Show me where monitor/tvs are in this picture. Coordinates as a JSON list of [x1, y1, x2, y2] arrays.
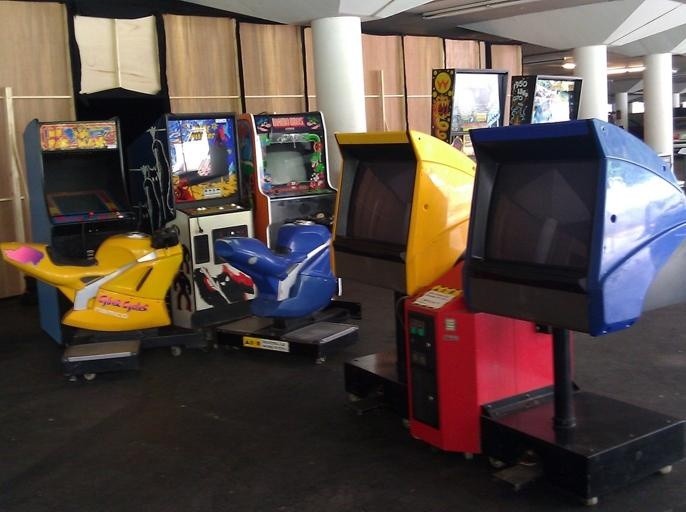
[[484, 157, 598, 272], [266, 142, 310, 185], [168, 118, 240, 204], [451, 72, 503, 132], [52, 192, 111, 217], [531, 79, 576, 126], [346, 158, 416, 247]]
